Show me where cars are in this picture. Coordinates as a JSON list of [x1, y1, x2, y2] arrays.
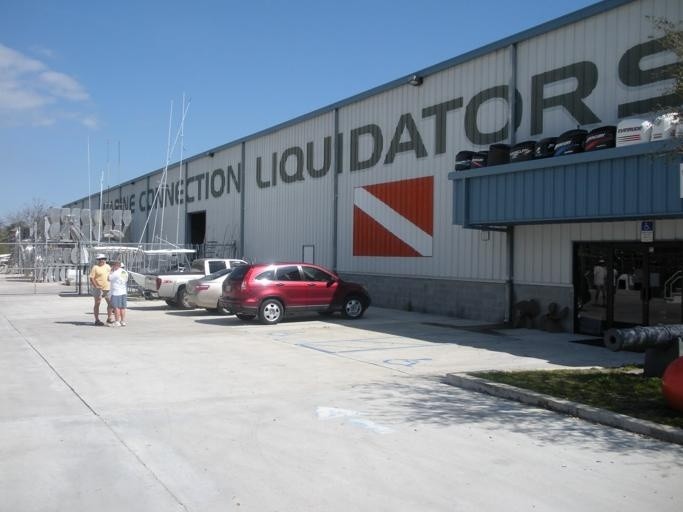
[[188, 266, 237, 316], [219, 261, 373, 325]]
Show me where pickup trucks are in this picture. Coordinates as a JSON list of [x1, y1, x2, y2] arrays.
[[144, 258, 248, 309]]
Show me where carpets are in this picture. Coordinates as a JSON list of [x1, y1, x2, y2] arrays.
[[569, 339, 646, 353]]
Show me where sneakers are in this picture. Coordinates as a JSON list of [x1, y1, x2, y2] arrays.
[[94, 320, 104, 326], [107, 318, 127, 327]]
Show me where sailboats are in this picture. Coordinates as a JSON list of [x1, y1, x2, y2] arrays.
[[127, 91, 197, 300]]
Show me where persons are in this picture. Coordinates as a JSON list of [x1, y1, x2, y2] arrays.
[[89, 254, 114, 326], [108, 261, 128, 327], [593, 259, 619, 307]]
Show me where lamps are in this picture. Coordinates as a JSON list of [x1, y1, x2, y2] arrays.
[[206, 152, 214, 157], [408, 75, 423, 86]]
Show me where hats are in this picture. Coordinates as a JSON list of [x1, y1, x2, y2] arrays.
[[96, 254, 107, 260]]
